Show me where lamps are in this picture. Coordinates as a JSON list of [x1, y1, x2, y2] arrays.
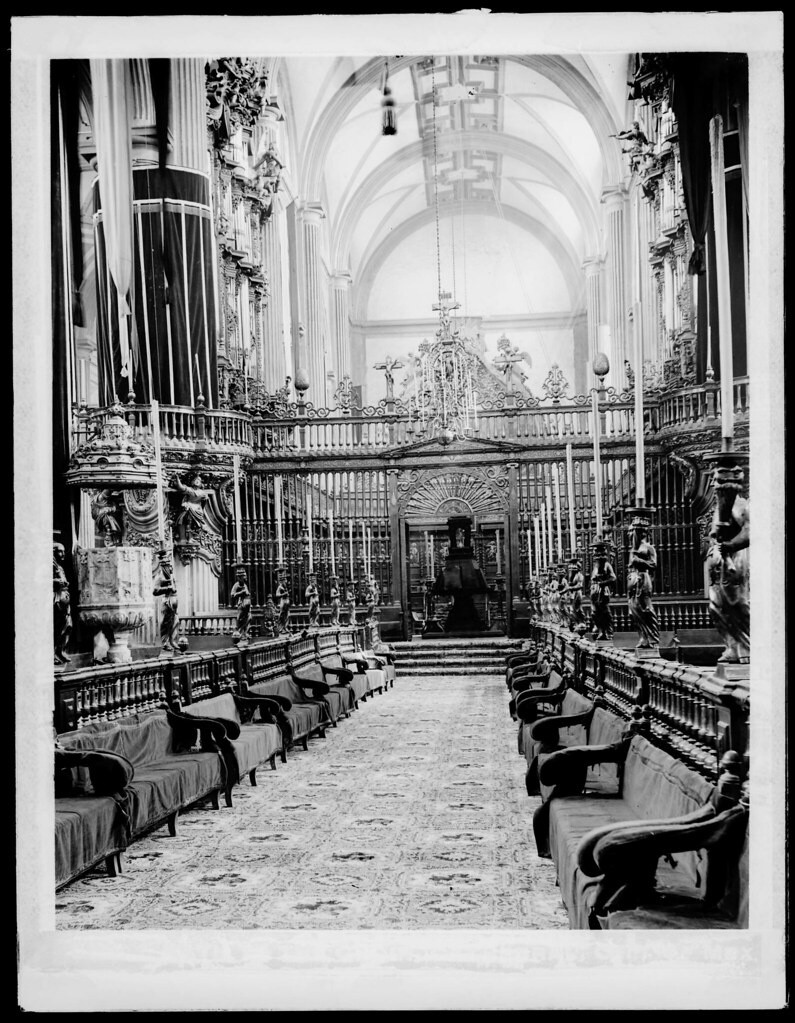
[[407, 56, 480, 445]]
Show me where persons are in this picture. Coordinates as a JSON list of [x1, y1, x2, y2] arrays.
[[625, 519, 662, 650], [90, 490, 124, 548], [276, 575, 291, 634], [52, 543, 74, 664], [588, 547, 620, 640], [264, 599, 277, 633], [229, 569, 253, 640], [525, 569, 572, 628], [173, 472, 210, 544], [616, 121, 650, 154], [705, 465, 751, 666], [303, 573, 381, 627], [564, 558, 589, 633], [151, 551, 182, 651], [255, 140, 288, 180]]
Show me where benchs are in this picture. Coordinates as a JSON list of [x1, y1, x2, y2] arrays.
[[505, 641, 748, 929], [54, 642, 397, 908]]
